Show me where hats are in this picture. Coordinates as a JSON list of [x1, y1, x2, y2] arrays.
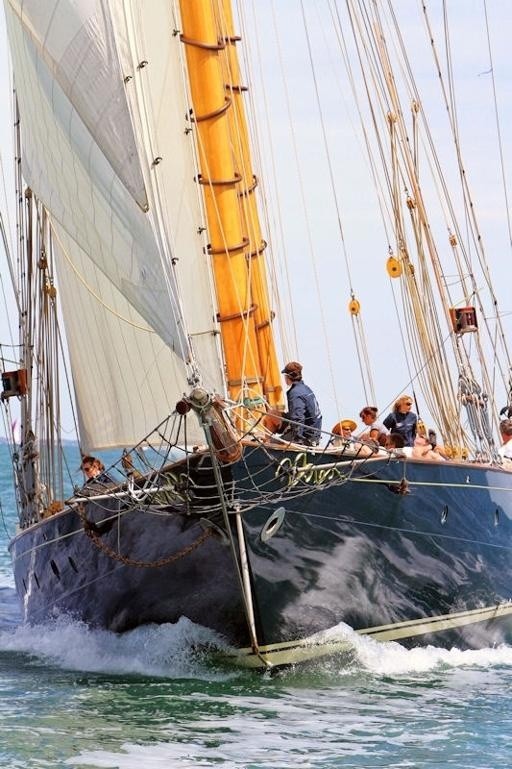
[[281, 362, 303, 374], [332, 420, 357, 433]]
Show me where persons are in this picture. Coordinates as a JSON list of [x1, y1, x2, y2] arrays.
[[77, 455, 126, 500], [268, 361, 322, 448], [495, 419, 511, 463], [329, 395, 416, 458]]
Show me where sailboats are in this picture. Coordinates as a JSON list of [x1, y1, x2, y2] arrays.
[[0, 0, 512, 679]]
